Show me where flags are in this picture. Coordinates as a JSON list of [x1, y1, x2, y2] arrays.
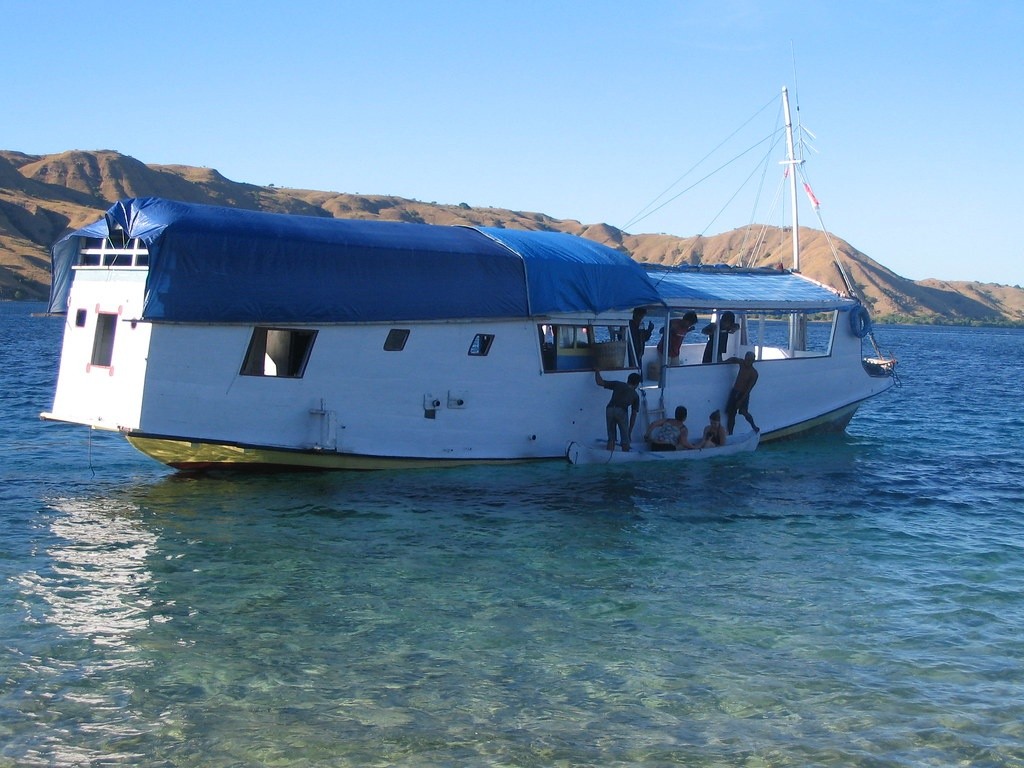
[[800, 179, 822, 207]]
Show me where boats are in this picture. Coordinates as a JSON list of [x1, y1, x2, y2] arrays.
[[39, 84, 896, 472], [565, 430, 759, 464]]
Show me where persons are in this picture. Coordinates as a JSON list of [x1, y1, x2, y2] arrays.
[[618, 307, 654, 385], [725, 351, 759, 436], [692, 409, 725, 448], [701, 311, 740, 363], [594, 367, 640, 452], [655, 310, 699, 388], [645, 405, 700, 451]]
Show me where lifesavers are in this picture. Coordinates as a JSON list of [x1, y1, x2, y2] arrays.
[[851, 305, 872, 338]]
